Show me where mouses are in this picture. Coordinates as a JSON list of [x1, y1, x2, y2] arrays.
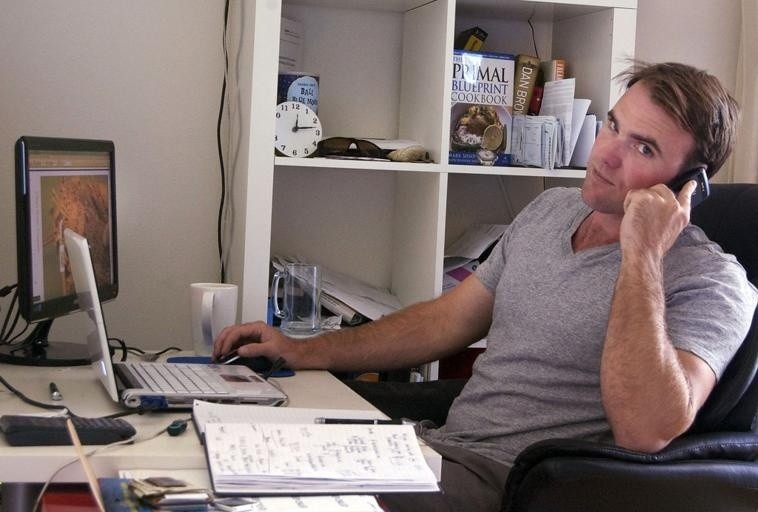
[[215, 344, 273, 374]]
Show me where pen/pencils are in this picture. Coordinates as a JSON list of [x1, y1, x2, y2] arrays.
[[315, 418, 405, 424]]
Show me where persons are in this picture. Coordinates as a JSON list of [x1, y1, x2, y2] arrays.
[[211, 62, 758, 512]]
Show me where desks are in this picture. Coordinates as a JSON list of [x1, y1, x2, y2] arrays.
[[1, 334, 395, 512]]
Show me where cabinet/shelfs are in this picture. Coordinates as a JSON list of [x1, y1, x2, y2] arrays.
[[234, 0, 644, 387]]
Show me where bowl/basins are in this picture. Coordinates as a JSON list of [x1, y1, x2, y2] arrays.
[[451, 104, 511, 154]]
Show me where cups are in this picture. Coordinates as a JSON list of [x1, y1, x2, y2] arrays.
[[267, 260, 328, 340], [187, 279, 242, 358]]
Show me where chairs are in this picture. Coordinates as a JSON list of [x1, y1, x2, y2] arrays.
[[350, 179, 755, 510]]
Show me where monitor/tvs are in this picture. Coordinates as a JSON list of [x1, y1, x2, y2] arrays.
[[0, 136, 120, 366]]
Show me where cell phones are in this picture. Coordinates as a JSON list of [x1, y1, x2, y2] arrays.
[[666, 168, 711, 212]]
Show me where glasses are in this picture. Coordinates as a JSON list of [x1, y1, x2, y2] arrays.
[[317, 137, 389, 161]]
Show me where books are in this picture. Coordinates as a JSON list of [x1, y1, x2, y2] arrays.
[[542, 59, 565, 82], [514, 55, 540, 116], [191, 399, 445, 496], [448, 49, 515, 167], [456, 28, 488, 51]]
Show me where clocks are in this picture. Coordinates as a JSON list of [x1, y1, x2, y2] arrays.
[[273, 97, 322, 158]]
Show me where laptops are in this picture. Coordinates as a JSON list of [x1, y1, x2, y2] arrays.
[[64, 227, 290, 410]]
